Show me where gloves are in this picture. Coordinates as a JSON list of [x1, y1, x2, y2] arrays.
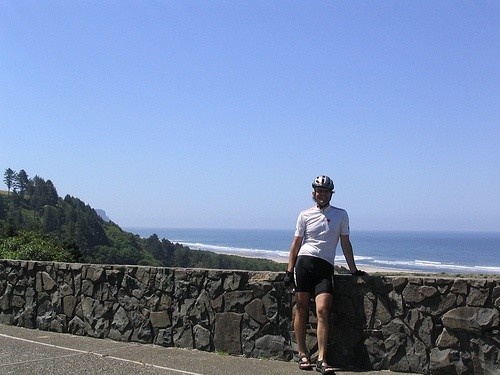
[[284, 270, 293, 286], [352, 270, 368, 277]]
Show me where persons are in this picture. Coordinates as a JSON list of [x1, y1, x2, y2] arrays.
[[284, 177, 369, 375]]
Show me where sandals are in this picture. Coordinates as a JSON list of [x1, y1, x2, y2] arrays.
[[315, 360, 334, 375], [297, 355, 312, 370]]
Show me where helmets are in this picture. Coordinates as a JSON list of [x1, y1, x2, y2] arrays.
[[312, 175, 335, 189]]
